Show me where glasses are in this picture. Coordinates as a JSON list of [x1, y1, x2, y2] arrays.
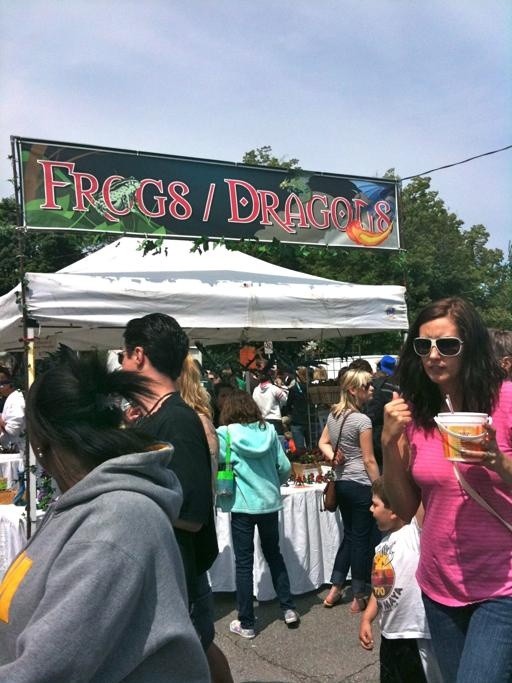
[[412, 336, 465, 358]]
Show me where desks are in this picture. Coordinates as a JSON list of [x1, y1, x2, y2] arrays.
[[1, 452, 29, 490], [1, 504, 44, 578], [206, 466, 352, 602]]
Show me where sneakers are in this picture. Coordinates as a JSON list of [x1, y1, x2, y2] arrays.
[[230, 619, 256, 638], [284, 609, 300, 623]]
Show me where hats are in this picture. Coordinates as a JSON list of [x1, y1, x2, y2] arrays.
[[380, 356, 396, 376]]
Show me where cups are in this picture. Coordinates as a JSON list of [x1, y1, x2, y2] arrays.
[[437, 411, 489, 463]]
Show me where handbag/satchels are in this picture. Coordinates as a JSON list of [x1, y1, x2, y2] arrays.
[[216, 472, 234, 498], [323, 481, 339, 512]]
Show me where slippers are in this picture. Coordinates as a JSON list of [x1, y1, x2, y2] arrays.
[[324, 594, 367, 613]]
